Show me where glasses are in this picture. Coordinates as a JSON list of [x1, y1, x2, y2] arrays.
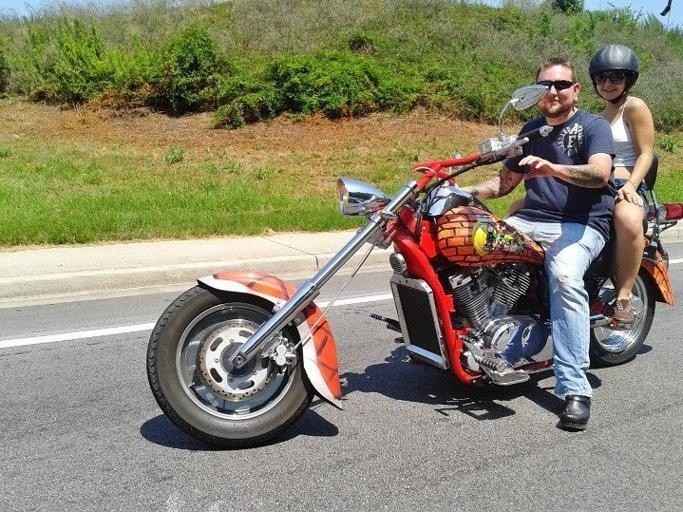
[[596, 71, 623, 85], [536, 79, 573, 90]]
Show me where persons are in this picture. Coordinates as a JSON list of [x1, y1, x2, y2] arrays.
[[587, 44, 655, 323], [455, 59, 616, 431]]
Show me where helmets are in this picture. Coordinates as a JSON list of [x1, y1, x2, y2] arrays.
[[591, 44, 640, 72]]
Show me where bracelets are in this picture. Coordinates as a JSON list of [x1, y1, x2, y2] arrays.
[[631, 181, 638, 189]]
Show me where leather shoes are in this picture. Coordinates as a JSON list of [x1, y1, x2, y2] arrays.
[[560, 394, 590, 429], [613, 288, 634, 322]]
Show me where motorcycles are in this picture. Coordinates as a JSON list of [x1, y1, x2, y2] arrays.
[[146, 84, 683, 450]]
